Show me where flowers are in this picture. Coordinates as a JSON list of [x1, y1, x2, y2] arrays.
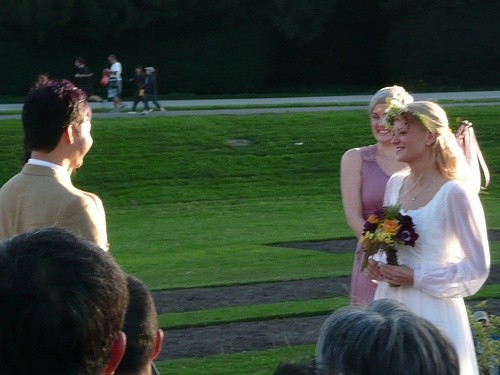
[[381, 97, 408, 128], [356, 202, 420, 287]]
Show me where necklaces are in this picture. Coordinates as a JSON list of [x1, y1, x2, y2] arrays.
[[407, 190, 423, 201]]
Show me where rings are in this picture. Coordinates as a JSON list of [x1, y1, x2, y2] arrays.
[[388, 275, 391, 280]]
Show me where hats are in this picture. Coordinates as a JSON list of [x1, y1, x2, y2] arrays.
[[144, 66, 155, 72]]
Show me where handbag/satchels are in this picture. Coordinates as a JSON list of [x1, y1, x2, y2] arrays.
[[100, 71, 110, 87]]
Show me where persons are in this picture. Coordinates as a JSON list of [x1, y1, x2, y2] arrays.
[[313, 296, 459, 375], [0, 226, 163, 375], [340, 84, 414, 304], [73, 57, 102, 102], [362, 100, 491, 375], [102, 54, 126, 113], [0, 73, 111, 252], [127, 65, 166, 115]]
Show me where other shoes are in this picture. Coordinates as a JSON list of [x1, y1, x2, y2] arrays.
[[159, 106, 166, 113], [128, 111, 137, 115], [141, 110, 149, 115]]
